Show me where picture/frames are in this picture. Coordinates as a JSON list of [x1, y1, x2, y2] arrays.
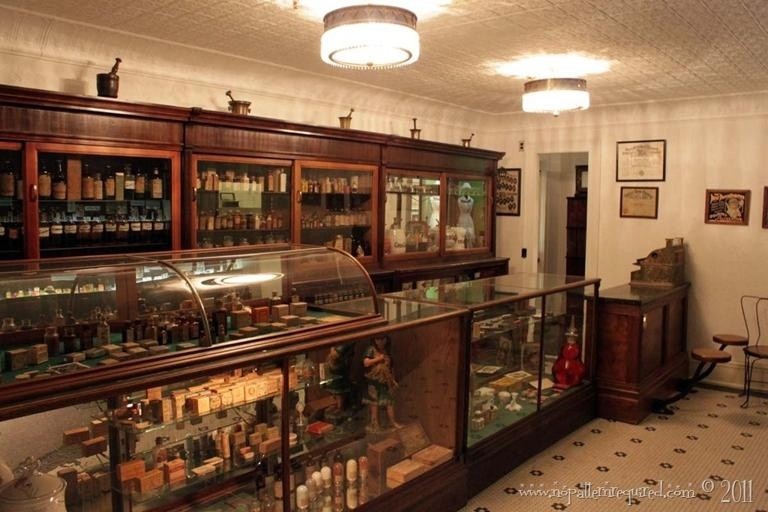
[[618, 186, 658, 219], [615, 139, 665, 180], [703, 188, 750, 224], [494, 167, 520, 217]]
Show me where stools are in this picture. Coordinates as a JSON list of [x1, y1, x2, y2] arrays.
[[648, 348, 731, 414], [676, 334, 748, 392]]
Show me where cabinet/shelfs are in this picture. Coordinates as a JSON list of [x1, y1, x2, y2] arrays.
[[2, 298, 471, 511], [381, 133, 504, 268], [0, 83, 186, 270], [185, 106, 382, 269], [383, 272, 605, 502], [592, 281, 691, 425]]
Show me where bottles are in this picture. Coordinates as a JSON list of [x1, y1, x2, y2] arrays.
[[256, 448, 372, 511], [197, 165, 286, 193], [198, 208, 282, 231], [0, 158, 23, 258], [302, 206, 367, 229], [326, 233, 364, 258], [384, 218, 407, 255], [0, 284, 299, 359], [301, 171, 359, 194], [0, 454, 68, 512], [39, 204, 169, 248], [37, 153, 163, 201], [470, 390, 518, 431]]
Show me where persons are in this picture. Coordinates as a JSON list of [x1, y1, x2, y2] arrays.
[[365, 334, 399, 428], [326, 341, 362, 411], [457, 182, 475, 238]]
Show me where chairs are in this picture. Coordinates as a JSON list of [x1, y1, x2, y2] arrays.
[[739, 294, 767, 408]]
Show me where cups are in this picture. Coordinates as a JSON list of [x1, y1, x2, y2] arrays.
[[390, 183, 434, 194]]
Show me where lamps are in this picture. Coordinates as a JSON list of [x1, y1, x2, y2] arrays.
[[319, 4, 419, 73], [520, 78, 588, 116]]
